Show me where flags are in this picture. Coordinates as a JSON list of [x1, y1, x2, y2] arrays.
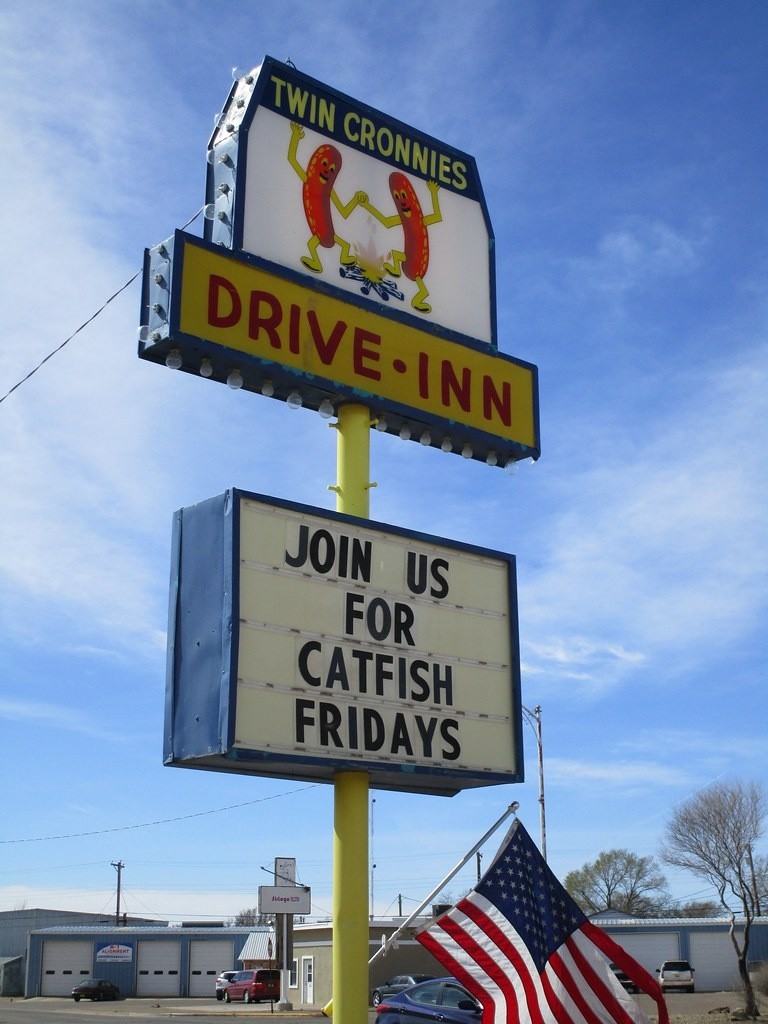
[[410, 819, 670, 1024]]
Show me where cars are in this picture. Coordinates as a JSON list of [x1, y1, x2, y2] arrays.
[[656, 960, 697, 993], [607, 962, 640, 993], [370, 973, 484, 1024], [71, 979, 120, 1002]]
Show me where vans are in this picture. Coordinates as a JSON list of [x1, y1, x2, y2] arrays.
[[215, 968, 281, 1003]]
